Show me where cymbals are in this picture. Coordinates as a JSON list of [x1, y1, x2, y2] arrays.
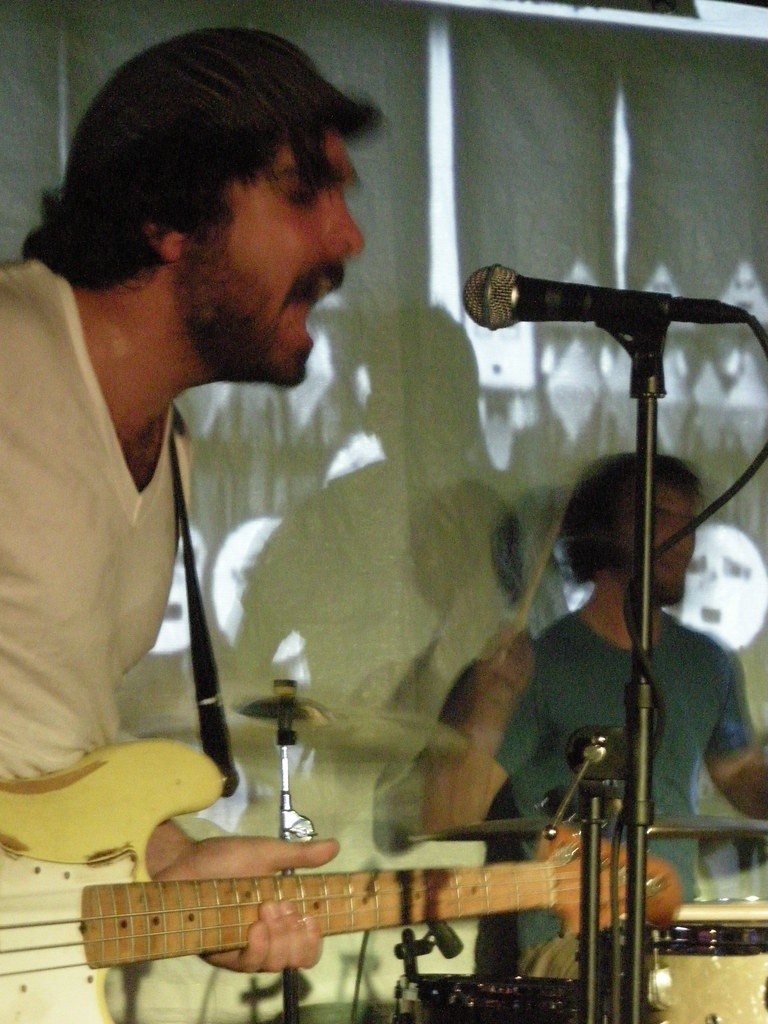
[[405, 807, 768, 843], [231, 695, 471, 762]]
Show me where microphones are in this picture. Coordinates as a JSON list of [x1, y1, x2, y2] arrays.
[[428, 921, 465, 960], [463, 263, 749, 330]]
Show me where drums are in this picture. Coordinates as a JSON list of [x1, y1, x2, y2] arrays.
[[417, 971, 586, 1023], [600, 898, 768, 1024]]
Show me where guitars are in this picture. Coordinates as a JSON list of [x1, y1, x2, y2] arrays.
[[1, 736, 680, 1024]]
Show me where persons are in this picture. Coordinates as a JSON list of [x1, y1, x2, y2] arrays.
[[1, 26, 381, 975], [424, 453, 768, 979]]
